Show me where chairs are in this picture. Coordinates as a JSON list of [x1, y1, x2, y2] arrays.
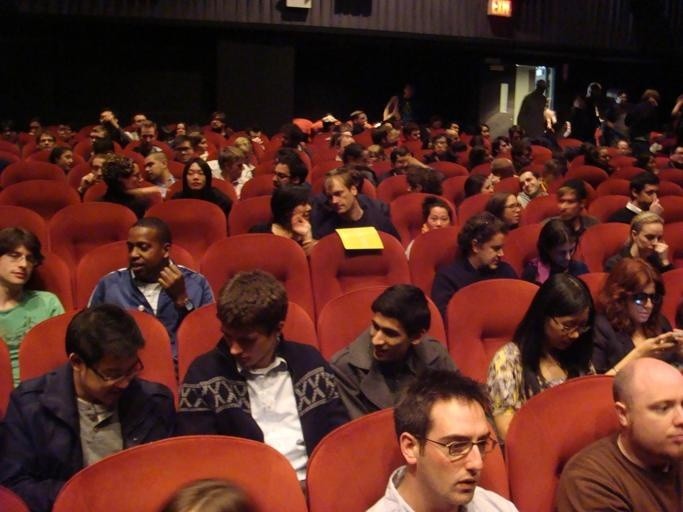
[[0, 124, 683, 511]]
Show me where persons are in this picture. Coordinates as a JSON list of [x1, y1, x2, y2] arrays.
[[363, 367, 523, 512], [592, 255, 682, 375], [0, 303, 175, 511], [330, 281, 459, 419], [176, 270, 350, 495], [522, 217, 589, 282], [427, 211, 523, 311], [601, 211, 675, 274], [553, 354, 682, 512], [84, 214, 214, 363], [1, 79, 682, 258], [0, 225, 66, 386], [156, 476, 255, 511], [483, 271, 597, 450]]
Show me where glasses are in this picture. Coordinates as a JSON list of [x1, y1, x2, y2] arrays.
[[422, 438, 496, 461], [88, 357, 144, 386], [623, 293, 660, 306], [551, 316, 588, 333]]
